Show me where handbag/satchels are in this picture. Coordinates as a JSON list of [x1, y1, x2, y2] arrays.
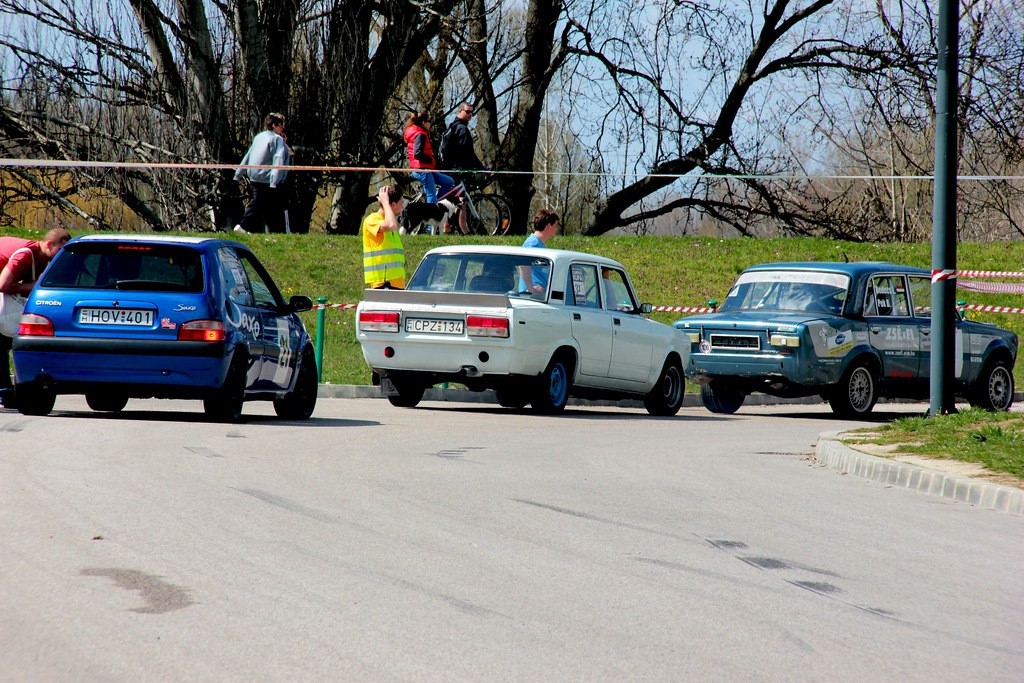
[[0, 248, 40, 338]]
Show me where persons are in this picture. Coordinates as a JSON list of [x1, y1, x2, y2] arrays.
[[438, 101, 495, 235], [0, 227, 72, 410], [233, 112, 294, 234], [517, 208, 560, 301], [362, 183, 406, 384], [403, 109, 454, 235]]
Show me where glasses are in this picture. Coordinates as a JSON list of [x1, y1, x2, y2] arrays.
[[426, 121, 432, 123], [462, 109, 472, 114]]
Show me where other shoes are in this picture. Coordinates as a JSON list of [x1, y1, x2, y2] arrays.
[[0, 400, 19, 413], [234, 224, 251, 234]]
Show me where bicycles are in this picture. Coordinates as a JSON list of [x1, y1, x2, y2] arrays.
[[379, 165, 514, 236]]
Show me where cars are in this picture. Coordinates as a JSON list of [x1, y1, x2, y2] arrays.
[[13, 235, 318, 422], [674, 261, 1020, 419], [356, 245, 691, 418]]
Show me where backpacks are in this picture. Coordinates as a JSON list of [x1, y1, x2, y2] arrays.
[[438, 122, 459, 165]]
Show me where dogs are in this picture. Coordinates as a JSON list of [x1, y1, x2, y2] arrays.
[[398, 196, 463, 235]]
